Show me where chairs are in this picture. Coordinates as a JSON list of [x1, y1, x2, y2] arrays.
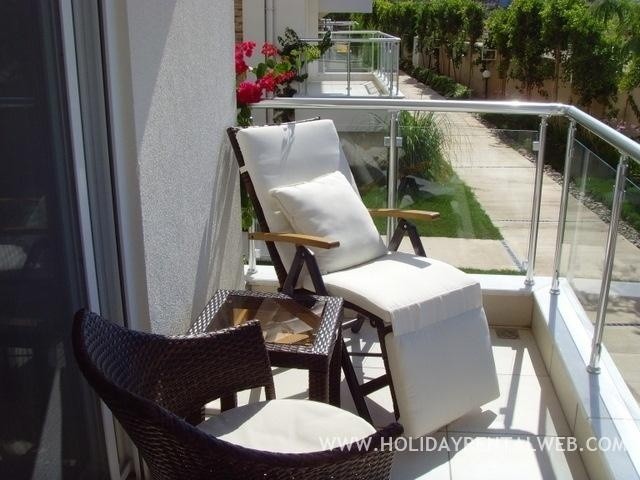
[[72, 304, 405, 478], [227, 115, 492, 426]]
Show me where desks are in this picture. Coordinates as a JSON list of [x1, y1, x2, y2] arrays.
[[190, 286, 343, 414]]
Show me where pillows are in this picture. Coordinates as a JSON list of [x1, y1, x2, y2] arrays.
[[265, 170, 390, 277]]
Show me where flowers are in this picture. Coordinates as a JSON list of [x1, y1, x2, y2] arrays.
[[235, 27, 335, 109]]
